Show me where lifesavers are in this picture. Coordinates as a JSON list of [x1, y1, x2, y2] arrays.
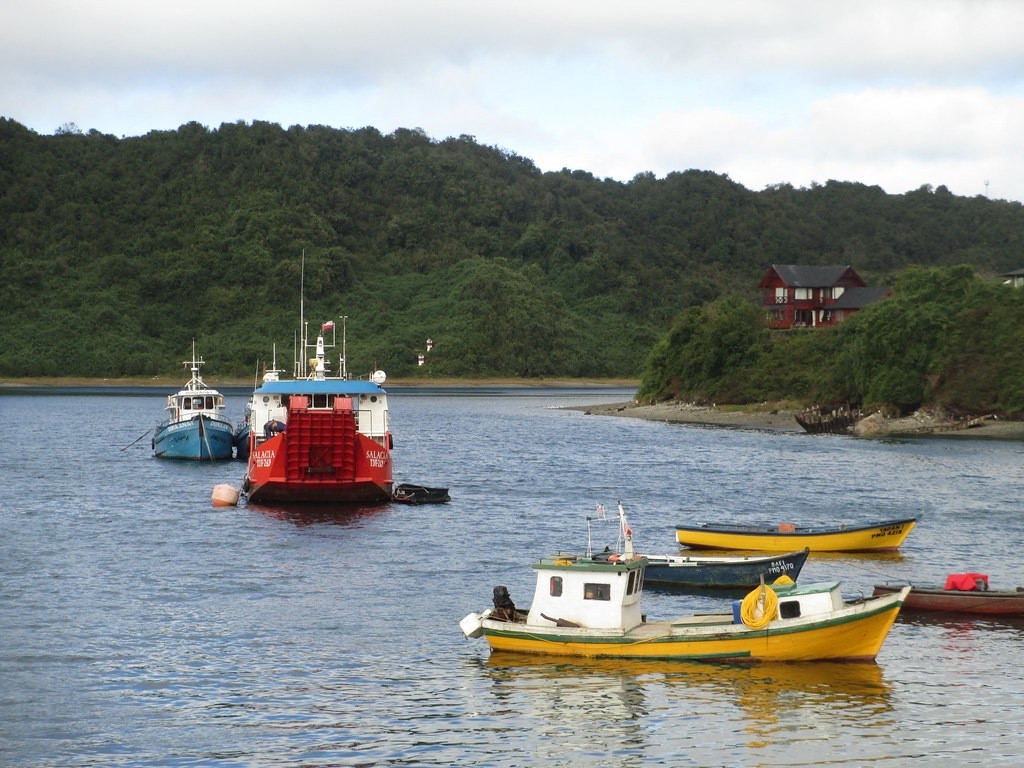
[[609, 554, 642, 563], [389, 434, 393, 449], [152, 438, 154, 449]]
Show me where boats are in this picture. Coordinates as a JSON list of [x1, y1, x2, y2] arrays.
[[584, 499, 812, 592], [232, 357, 260, 462], [393, 482, 453, 504], [872, 572, 1024, 618], [459, 500, 914, 665], [673, 509, 927, 553], [152, 338, 234, 461], [241, 249, 394, 503]]
